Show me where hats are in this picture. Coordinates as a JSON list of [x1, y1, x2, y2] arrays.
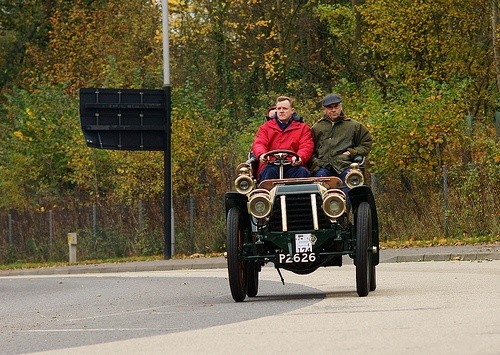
[[322, 94, 341, 106]]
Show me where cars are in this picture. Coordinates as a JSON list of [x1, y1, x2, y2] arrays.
[[223, 150, 382, 303]]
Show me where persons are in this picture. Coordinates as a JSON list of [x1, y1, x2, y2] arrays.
[[268, 107, 279, 119], [252, 95, 315, 183], [306, 93, 373, 213]]
